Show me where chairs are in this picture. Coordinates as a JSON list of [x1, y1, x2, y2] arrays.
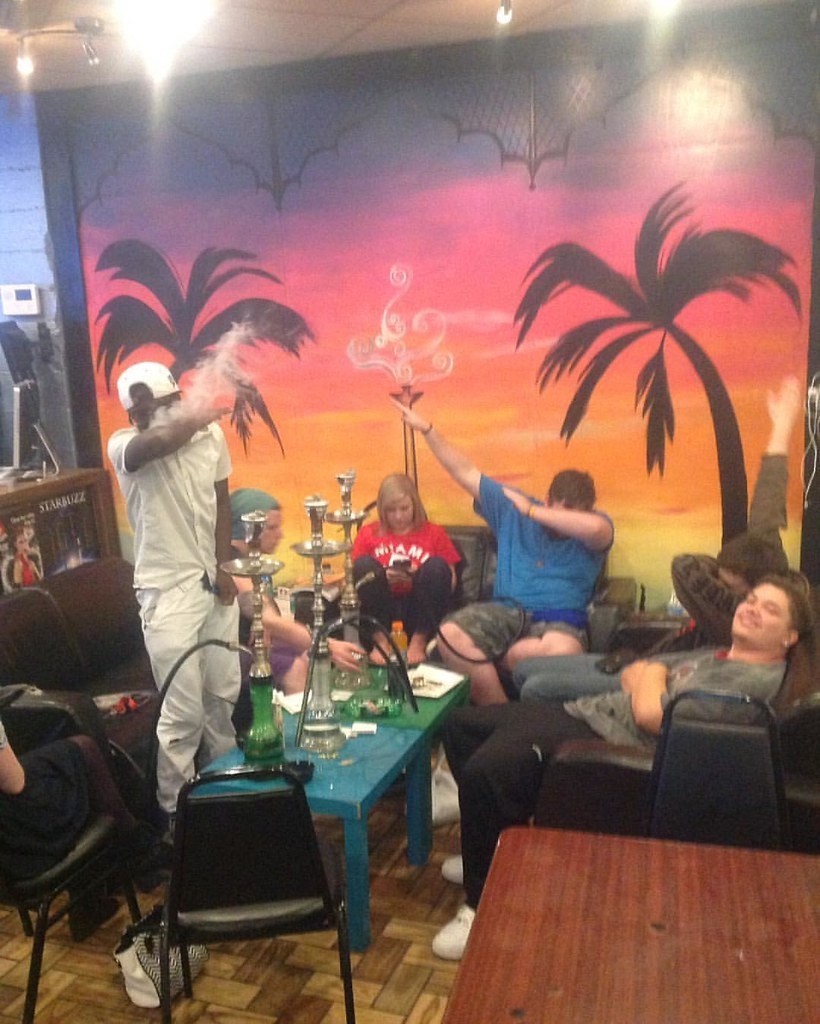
[[0, 700, 143, 1024], [161, 764, 356, 1024]]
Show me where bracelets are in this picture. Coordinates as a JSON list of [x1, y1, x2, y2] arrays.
[[423, 424, 432, 434], [528, 505, 535, 519]]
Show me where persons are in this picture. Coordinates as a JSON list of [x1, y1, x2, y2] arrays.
[[109, 363, 244, 816], [8, 527, 40, 586], [0, 721, 174, 940], [351, 472, 463, 665], [230, 487, 366, 694], [512, 373, 803, 703], [432, 574, 813, 961], [390, 399, 614, 707]]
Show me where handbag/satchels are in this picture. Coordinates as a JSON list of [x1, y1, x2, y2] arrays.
[[111, 903, 209, 1006]]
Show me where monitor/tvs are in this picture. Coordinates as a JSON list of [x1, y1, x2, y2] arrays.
[[12, 379, 61, 483]]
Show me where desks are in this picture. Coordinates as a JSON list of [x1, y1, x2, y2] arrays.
[[0, 466, 121, 595], [439, 827, 820, 1024], [188, 667, 472, 954]]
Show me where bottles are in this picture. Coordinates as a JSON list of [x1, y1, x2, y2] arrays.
[[667, 587, 684, 618], [339, 695, 402, 718], [387, 621, 406, 701]]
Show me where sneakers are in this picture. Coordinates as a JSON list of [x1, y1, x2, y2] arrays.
[[431, 904, 478, 962], [441, 853, 468, 885]]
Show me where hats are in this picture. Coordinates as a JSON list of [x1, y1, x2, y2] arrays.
[[117, 364, 181, 411]]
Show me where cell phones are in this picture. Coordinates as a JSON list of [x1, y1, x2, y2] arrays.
[[595, 648, 637, 674], [394, 559, 411, 571]]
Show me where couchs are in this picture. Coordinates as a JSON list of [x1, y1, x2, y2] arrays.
[[294, 524, 638, 700], [529, 612, 820, 858], [0, 555, 167, 891]]
